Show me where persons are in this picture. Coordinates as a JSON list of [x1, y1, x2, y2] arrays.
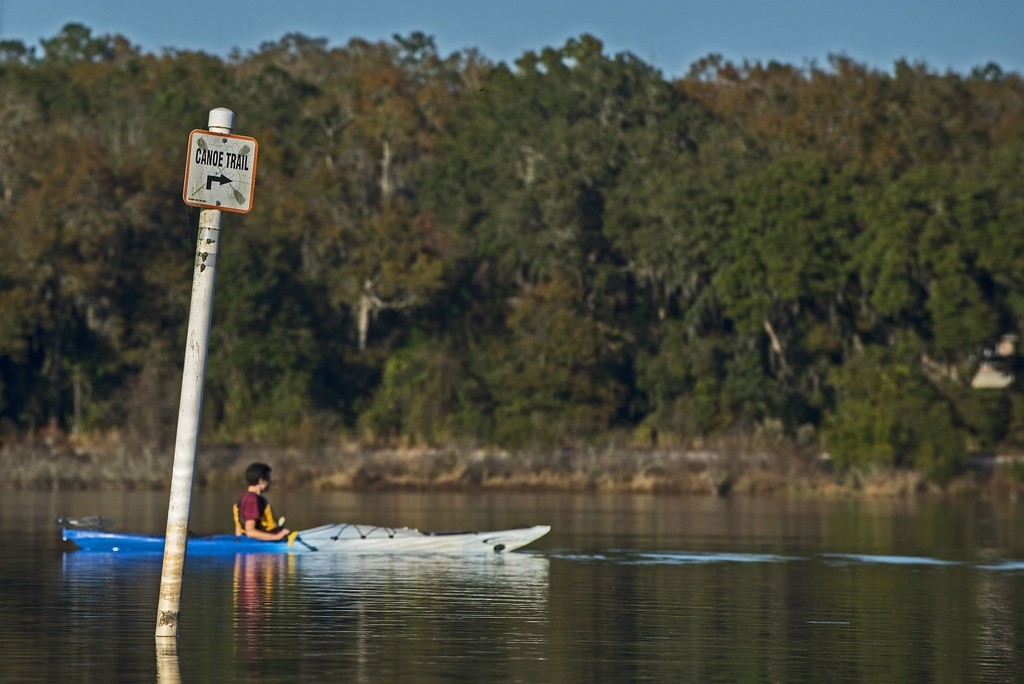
[[233, 462, 290, 540]]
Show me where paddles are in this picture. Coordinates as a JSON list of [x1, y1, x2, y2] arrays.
[[292, 531, 321, 553]]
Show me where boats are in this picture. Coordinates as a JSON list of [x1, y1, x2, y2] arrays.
[[59, 523, 551, 557]]
